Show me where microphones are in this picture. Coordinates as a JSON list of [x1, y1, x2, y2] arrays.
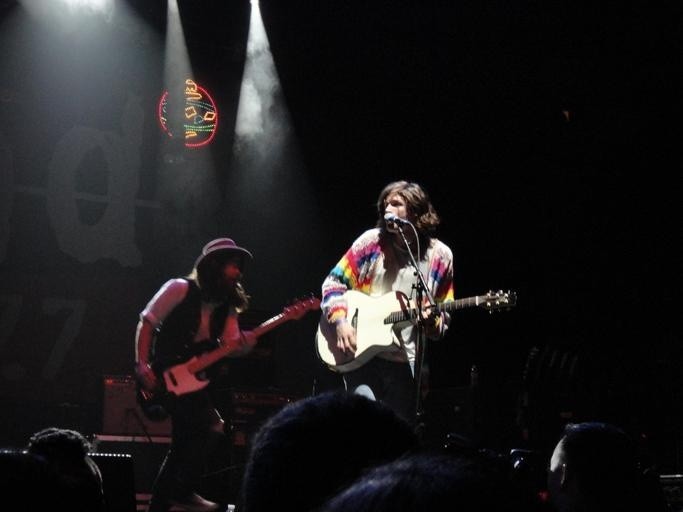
[[383, 212, 410, 225]]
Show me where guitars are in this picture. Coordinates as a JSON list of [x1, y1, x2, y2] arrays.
[[135, 292, 321, 422], [315, 286, 518, 373]]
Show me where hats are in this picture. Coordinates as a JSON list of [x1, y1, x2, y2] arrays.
[[195, 237, 253, 276]]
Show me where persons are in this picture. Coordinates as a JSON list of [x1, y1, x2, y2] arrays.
[[0, 424, 108, 511], [320, 180, 457, 413], [134, 237, 256, 512], [236, 390, 645, 511]]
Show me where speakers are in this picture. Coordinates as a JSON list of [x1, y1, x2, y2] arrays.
[[0, 449, 136, 512], [102, 375, 174, 436]]
